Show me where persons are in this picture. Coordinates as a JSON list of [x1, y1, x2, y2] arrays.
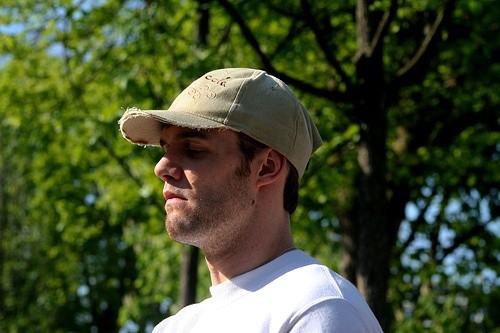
[[118, 68, 384, 333]]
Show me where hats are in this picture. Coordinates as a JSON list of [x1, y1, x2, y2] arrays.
[[118, 67, 323, 178]]
